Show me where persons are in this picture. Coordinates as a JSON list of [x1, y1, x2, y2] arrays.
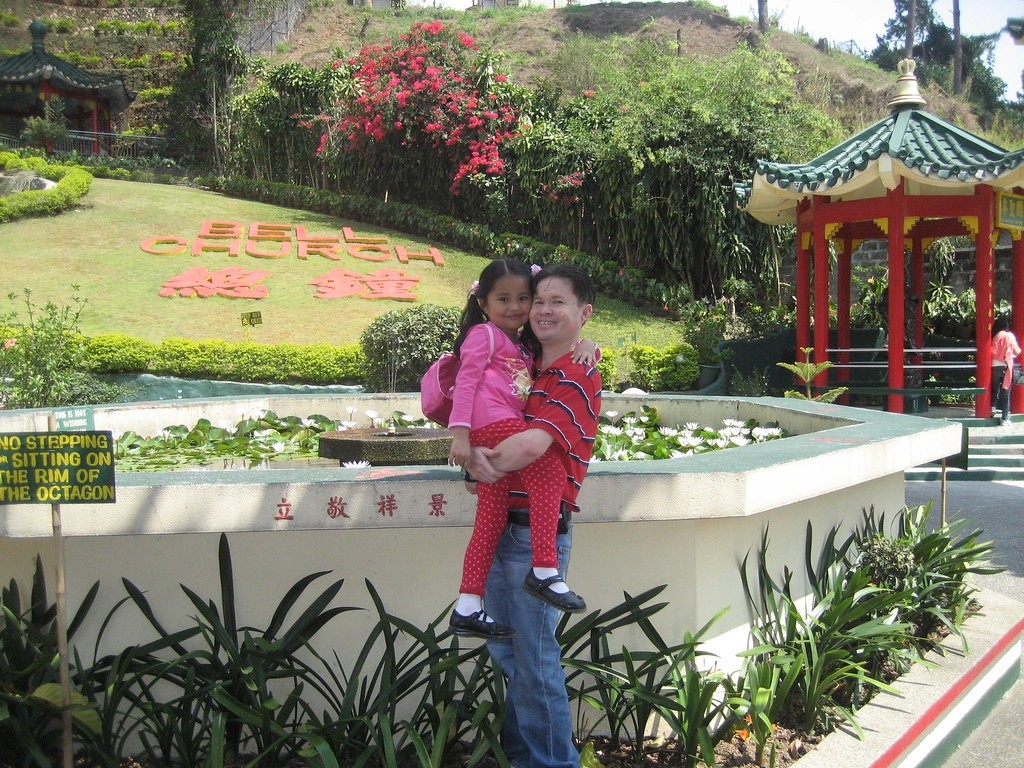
[[449, 257, 602, 640], [479, 263, 601, 768], [990, 316, 1021, 426]]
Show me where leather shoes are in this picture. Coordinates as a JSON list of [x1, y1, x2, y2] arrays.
[[448, 609, 516, 639], [524, 567, 587, 613]]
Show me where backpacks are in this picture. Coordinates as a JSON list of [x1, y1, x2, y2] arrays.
[[421, 324, 494, 427]]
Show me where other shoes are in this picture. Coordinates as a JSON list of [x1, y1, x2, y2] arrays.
[[999, 417, 1012, 425], [992, 407, 996, 416]]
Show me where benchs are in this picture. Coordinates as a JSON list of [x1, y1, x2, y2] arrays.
[[798, 380, 985, 411]]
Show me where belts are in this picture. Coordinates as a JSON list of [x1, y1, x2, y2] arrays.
[[508, 511, 571, 527]]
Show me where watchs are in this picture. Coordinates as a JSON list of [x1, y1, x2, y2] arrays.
[[461, 468, 478, 483]]
